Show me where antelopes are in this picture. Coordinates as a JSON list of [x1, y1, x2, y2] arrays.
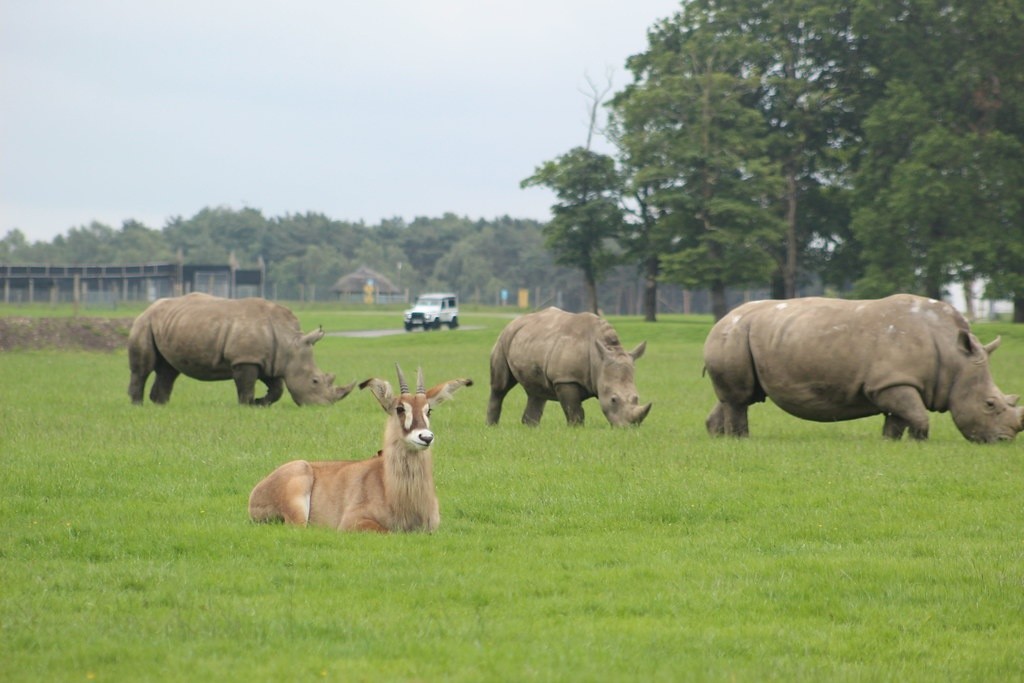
[[248, 361, 474, 534]]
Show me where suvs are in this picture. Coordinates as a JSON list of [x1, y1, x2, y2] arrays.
[[404, 292, 459, 331]]
